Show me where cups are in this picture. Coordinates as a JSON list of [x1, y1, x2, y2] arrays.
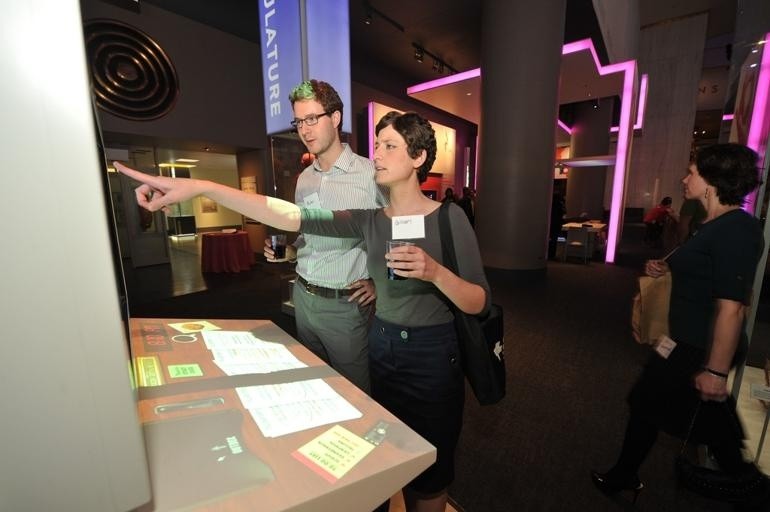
[[385, 240, 415, 281], [271, 234, 288, 260]]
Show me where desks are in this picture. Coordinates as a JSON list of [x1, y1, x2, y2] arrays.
[[561, 222, 607, 265], [201, 230, 256, 273]]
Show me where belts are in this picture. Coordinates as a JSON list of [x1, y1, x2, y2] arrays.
[[295, 273, 357, 299]]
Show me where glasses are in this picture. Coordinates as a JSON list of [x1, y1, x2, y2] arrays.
[[290, 110, 329, 128]]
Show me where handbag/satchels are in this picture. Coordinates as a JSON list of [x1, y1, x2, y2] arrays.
[[438, 199, 506, 407], [630, 244, 679, 345]]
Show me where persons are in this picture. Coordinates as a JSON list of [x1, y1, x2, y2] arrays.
[[264, 80, 389, 396], [641, 196, 672, 249], [112, 110, 493, 512], [592, 142, 765, 507], [458, 187, 474, 229], [469, 188, 476, 215], [678, 198, 707, 242], [548, 190, 562, 260], [441, 188, 454, 202]]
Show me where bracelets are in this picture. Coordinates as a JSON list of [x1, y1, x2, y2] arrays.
[[706, 368, 728, 379]]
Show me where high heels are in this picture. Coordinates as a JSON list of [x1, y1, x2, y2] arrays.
[[591, 472, 643, 505]]
